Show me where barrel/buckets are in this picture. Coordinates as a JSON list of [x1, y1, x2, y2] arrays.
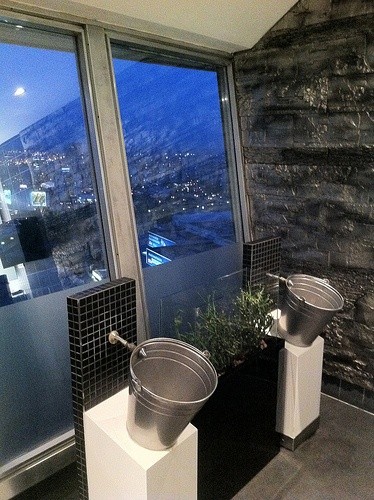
[[125, 338, 219, 450], [277, 273, 345, 348]]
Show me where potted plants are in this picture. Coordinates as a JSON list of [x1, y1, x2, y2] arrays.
[[169, 281, 284, 472]]
